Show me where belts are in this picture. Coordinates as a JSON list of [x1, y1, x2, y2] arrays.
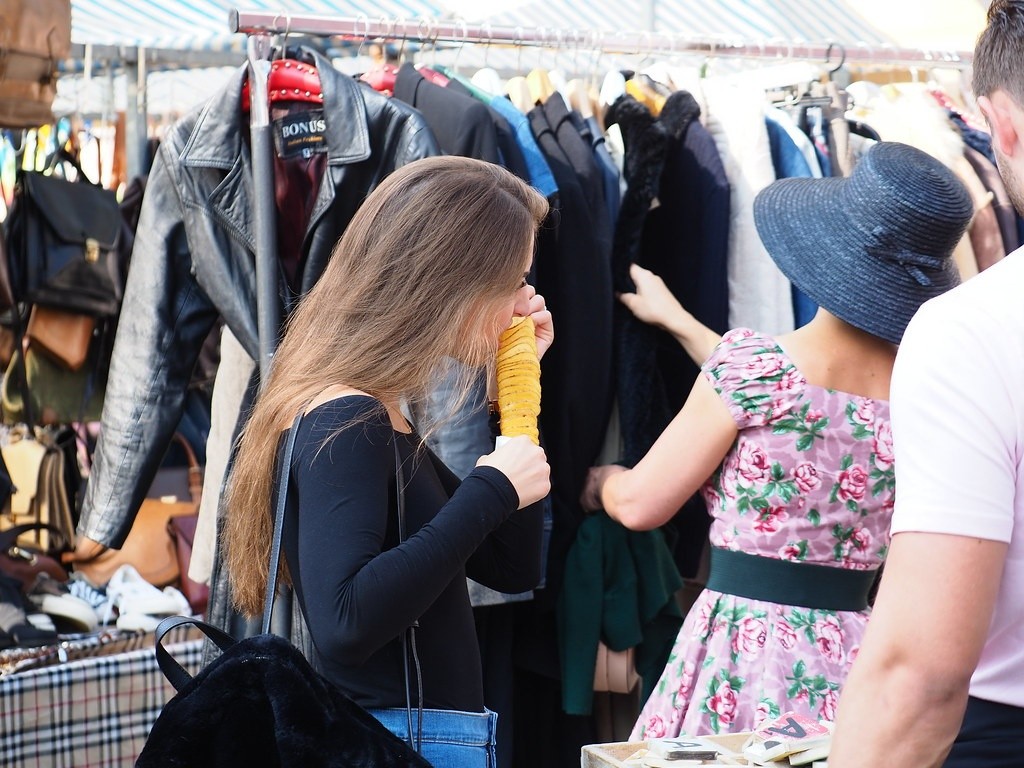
[[704, 546, 881, 612]]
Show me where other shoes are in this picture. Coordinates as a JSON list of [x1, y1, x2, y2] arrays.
[[28, 593, 97, 634], [2, 614, 60, 648], [68, 581, 119, 624], [117, 586, 191, 631], [104, 563, 179, 614]]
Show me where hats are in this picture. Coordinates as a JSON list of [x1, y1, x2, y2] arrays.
[[753, 141, 974, 345]]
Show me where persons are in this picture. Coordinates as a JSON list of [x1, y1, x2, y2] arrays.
[[577, 137, 974, 742], [817, 0, 1024, 768], [221, 154, 555, 768]]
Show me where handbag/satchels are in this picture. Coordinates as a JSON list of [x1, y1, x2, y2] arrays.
[[0, 425, 77, 554], [7, 126, 120, 316], [36, 347, 110, 424], [21, 306, 92, 369], [167, 512, 209, 614], [0, 233, 14, 313], [71, 425, 201, 591], [128, 615, 437, 768], [119, 140, 220, 392], [1, 350, 28, 425]]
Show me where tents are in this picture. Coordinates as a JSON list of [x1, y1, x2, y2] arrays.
[[61, 0, 901, 184]]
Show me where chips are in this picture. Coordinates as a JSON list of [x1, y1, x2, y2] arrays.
[[498, 316, 541, 445]]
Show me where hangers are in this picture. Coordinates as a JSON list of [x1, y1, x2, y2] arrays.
[[274, 12, 964, 118]]
